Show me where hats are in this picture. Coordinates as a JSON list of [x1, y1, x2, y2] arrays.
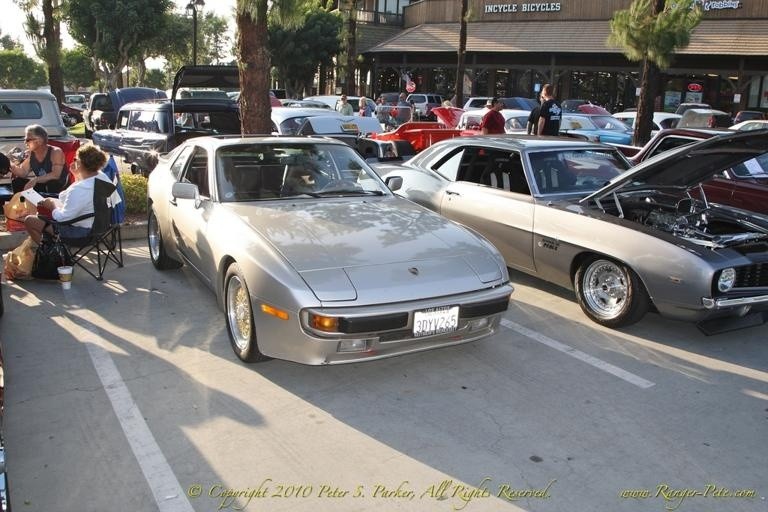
[[341, 95, 346, 99]]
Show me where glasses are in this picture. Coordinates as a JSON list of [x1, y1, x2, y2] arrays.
[[73, 158, 79, 162], [24, 138, 39, 142]]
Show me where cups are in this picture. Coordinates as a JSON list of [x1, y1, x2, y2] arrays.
[[57, 266, 73, 290]]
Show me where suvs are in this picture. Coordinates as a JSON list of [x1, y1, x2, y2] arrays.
[[90, 66, 240, 164]]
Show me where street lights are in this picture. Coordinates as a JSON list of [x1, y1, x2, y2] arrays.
[[185, 0, 207, 66]]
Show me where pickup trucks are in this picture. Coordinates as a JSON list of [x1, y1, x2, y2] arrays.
[[604, 129, 767, 212], [0, 90, 77, 151], [65, 95, 88, 109]]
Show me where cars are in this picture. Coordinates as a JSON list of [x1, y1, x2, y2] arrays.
[[272, 92, 735, 160], [363, 134, 768, 331], [62, 103, 84, 121], [733, 111, 768, 124], [726, 120, 767, 132], [144, 134, 513, 366]]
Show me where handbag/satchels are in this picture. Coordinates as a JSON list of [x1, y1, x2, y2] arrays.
[[32, 241, 73, 279]]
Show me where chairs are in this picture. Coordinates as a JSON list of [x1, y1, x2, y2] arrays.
[[38, 167, 124, 281], [32, 137, 81, 196]]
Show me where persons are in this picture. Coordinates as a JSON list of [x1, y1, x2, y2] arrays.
[[375, 96, 390, 127], [24, 145, 113, 248], [537, 84, 562, 136], [481, 98, 506, 135], [358, 96, 372, 118], [527, 92, 546, 136], [336, 94, 353, 117], [10, 124, 71, 199], [397, 93, 414, 125]]
